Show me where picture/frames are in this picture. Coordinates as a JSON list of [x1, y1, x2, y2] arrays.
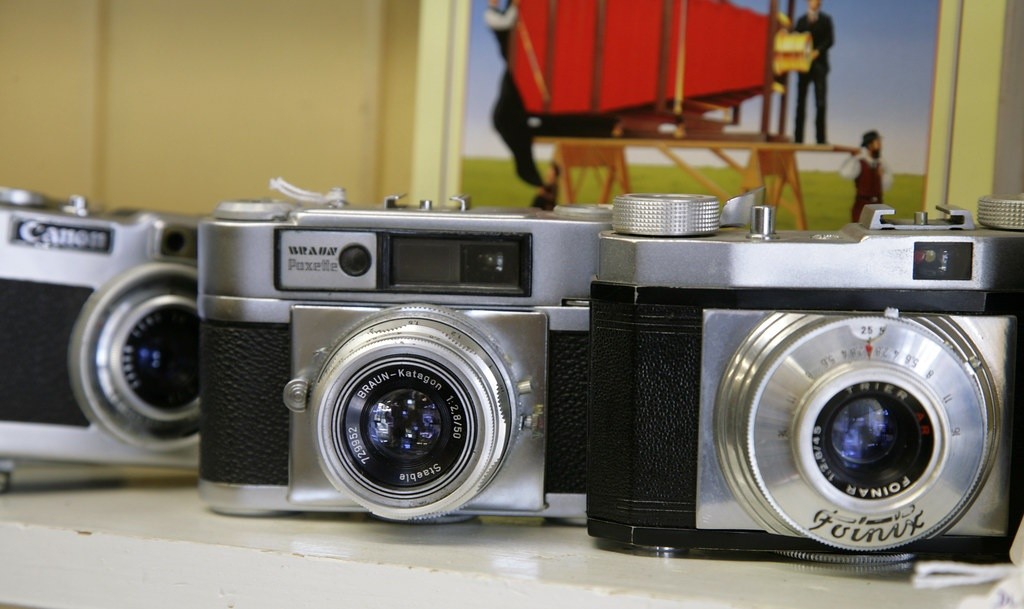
[[408, 0, 1004, 226]]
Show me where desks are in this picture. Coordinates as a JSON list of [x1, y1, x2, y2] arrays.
[[0, 465, 1001, 608]]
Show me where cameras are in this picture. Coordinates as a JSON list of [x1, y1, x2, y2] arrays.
[[0, 186, 202, 492], [196, 189, 614, 524], [586, 192, 1024, 566]]
